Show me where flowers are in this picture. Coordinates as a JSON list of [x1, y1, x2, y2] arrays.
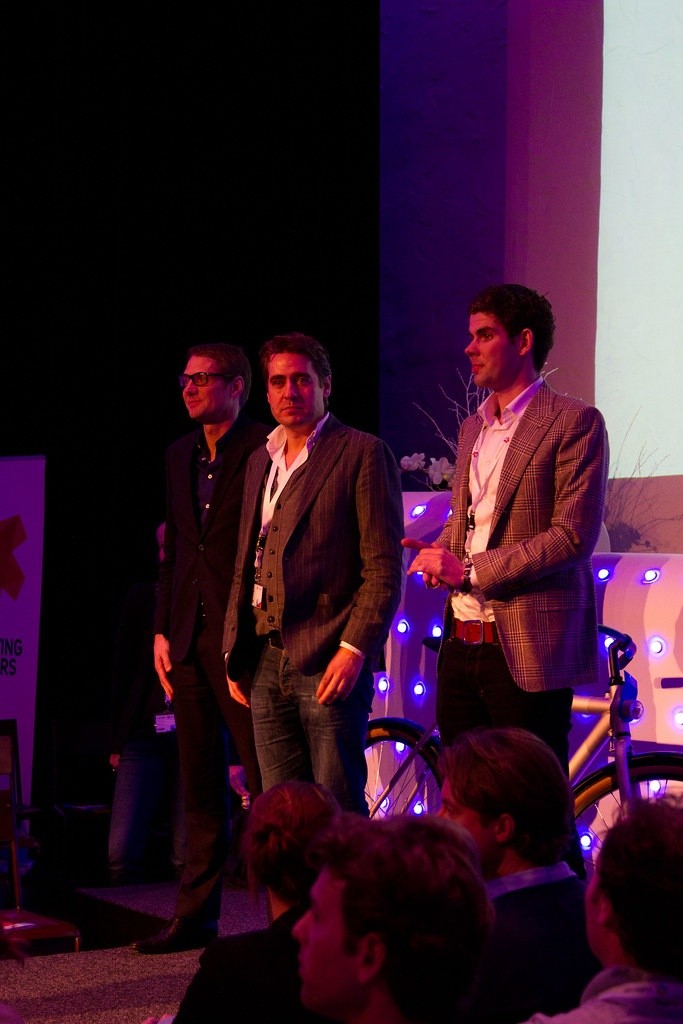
[[394, 449, 457, 489]]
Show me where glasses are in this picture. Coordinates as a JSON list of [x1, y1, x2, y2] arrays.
[[180, 372, 233, 389]]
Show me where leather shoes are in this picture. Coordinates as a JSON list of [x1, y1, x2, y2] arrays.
[[131, 915, 217, 953]]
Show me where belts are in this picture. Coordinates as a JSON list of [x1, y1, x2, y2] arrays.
[[261, 630, 284, 651], [450, 618, 497, 646]]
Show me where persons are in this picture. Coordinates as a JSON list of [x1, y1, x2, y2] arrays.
[[110, 667, 180, 887], [432, 728, 604, 1024], [290, 815, 496, 1024], [131, 343, 275, 951], [401, 284, 610, 1023], [170, 780, 343, 1024], [521, 800, 683, 1024], [220, 332, 405, 820]]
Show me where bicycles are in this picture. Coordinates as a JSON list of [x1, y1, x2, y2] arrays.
[[364, 625, 683, 882]]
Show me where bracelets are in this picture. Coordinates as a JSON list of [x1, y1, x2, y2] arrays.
[[461, 562, 474, 592]]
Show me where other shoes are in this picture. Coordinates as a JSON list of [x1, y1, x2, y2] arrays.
[[79, 861, 148, 890]]
